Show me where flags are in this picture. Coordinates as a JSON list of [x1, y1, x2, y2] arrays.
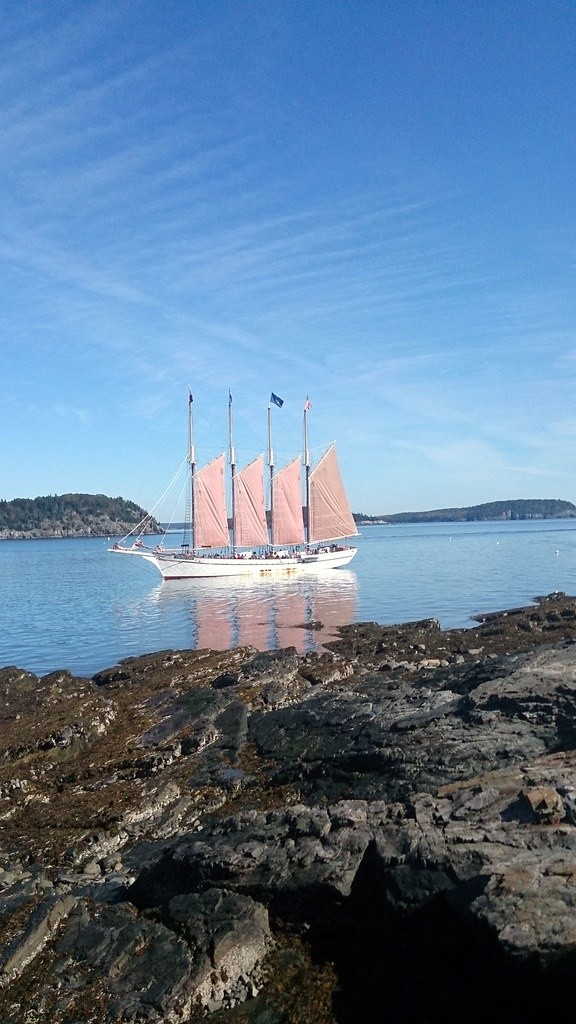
[[188, 387, 312, 412]]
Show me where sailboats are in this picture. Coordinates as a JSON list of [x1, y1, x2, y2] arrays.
[[104, 384, 364, 581]]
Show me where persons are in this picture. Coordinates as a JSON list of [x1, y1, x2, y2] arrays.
[[182, 543, 344, 562]]
